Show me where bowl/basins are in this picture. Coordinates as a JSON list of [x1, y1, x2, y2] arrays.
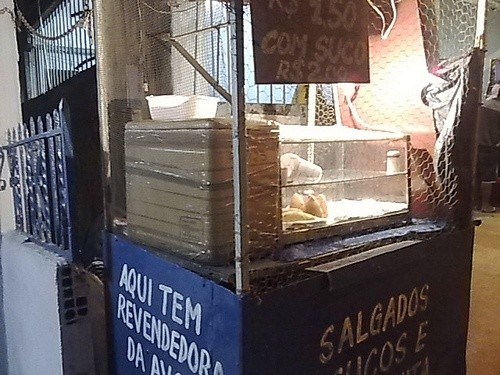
[[144, 94, 220, 119]]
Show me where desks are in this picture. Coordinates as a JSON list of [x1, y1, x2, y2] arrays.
[[94, 215, 481, 374]]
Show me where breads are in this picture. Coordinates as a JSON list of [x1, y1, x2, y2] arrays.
[[282, 207, 315, 222], [290, 193, 306, 210]]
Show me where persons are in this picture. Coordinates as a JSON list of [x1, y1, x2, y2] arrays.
[[472, 79, 500, 212]]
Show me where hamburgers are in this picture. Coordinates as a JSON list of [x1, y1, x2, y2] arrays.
[[303, 194, 328, 218]]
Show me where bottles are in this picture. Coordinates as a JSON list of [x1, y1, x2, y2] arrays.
[[387, 150, 400, 175]]
